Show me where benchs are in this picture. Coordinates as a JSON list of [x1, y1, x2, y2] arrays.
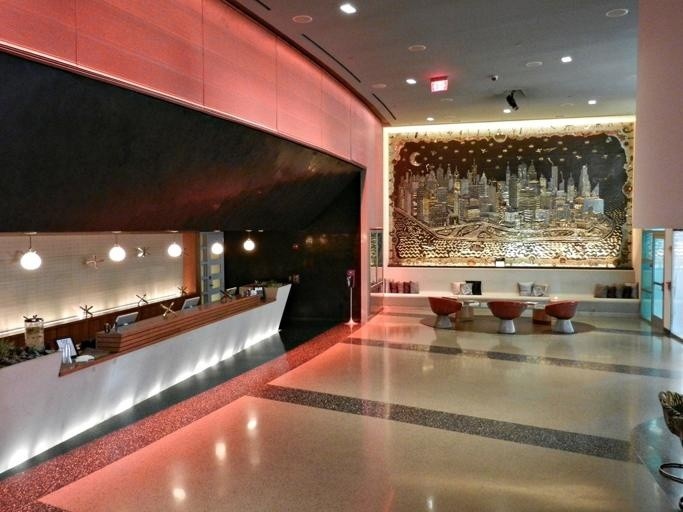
[[384, 290, 642, 319]]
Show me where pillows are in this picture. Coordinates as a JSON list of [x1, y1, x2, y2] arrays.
[[518, 281, 547, 297], [451, 280, 483, 295], [593, 282, 638, 298], [386, 278, 419, 294]]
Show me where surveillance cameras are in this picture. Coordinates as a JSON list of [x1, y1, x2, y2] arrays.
[[506, 94, 519, 111], [491, 75, 497, 81]]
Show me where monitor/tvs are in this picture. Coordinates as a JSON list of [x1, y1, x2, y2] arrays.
[[226, 287, 237, 296], [182, 297, 200, 310], [113, 312, 138, 328]]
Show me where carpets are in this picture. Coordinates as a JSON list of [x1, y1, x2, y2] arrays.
[[630, 416, 683, 512]]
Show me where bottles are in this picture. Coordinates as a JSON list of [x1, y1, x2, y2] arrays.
[[59, 339, 72, 369]]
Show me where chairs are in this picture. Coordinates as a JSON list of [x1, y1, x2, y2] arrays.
[[658, 390, 682, 482], [428, 297, 462, 328], [546, 300, 579, 334], [487, 301, 528, 334]]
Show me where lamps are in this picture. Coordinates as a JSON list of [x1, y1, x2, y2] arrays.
[[506, 91, 519, 113], [431, 79, 449, 92], [20, 235, 42, 270], [211, 230, 223, 254], [109, 231, 126, 262], [243, 230, 256, 251], [167, 231, 181, 257]]
